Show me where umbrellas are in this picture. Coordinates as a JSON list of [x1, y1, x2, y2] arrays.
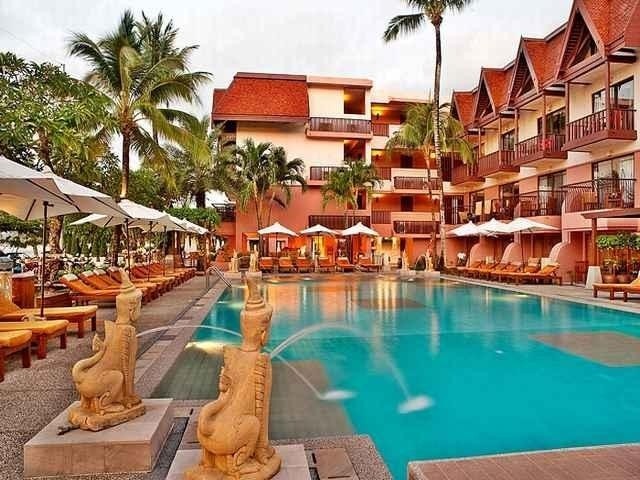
[[446, 216, 560, 273], [256, 221, 379, 257], [0, 155, 210, 316]]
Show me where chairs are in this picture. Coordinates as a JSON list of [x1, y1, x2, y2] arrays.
[[457, 176, 628, 224], [247, 246, 387, 275], [443, 250, 640, 305], [0, 248, 211, 384]]
